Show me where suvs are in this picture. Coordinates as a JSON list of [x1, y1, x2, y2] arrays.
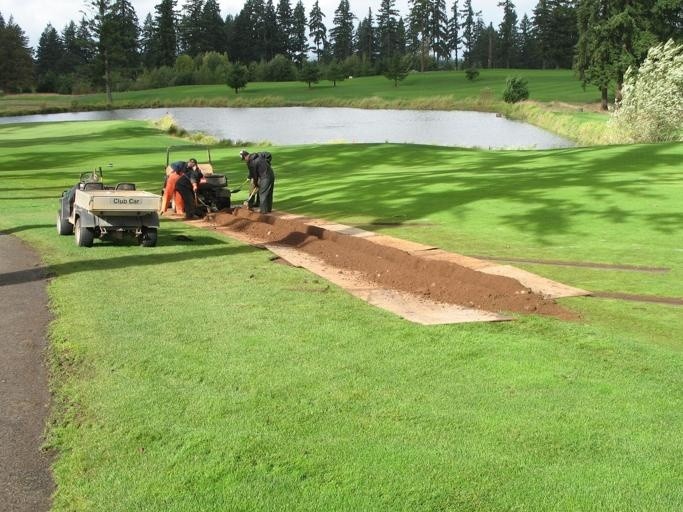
[[56, 167, 161, 246]]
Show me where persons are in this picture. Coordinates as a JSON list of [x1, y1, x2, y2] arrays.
[[240, 150, 274, 213], [159, 159, 196, 215], [174, 168, 206, 217]]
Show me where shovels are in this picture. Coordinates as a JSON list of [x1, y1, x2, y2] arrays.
[[230, 179, 248, 193]]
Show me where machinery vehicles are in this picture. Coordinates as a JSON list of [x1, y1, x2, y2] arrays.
[[160, 142, 232, 217]]
